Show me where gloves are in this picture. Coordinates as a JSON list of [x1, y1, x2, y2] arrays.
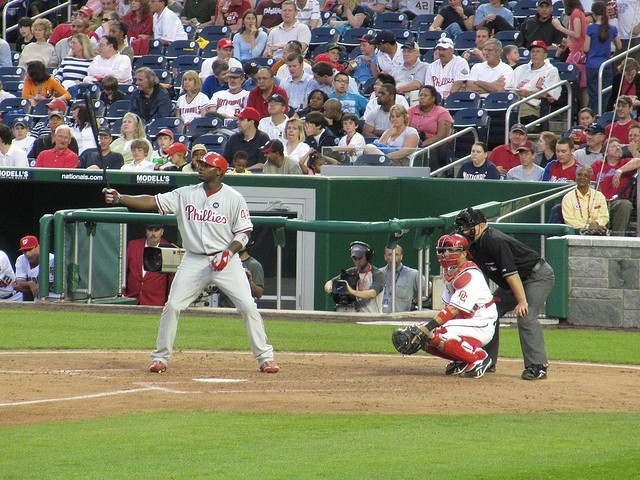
[[210, 249, 233, 271], [102, 188, 122, 204]]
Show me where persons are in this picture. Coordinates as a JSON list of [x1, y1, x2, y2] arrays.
[[379, 244, 432, 313], [453, 207, 555, 380], [324, 243, 387, 314], [126, 224, 175, 306], [592, 0, 639, 137], [0, 249, 23, 301], [561, 165, 610, 235], [592, 137, 640, 237], [13, 235, 54, 302], [0, 0, 592, 182], [218, 237, 265, 308], [102, 152, 279, 373], [411, 233, 498, 379]]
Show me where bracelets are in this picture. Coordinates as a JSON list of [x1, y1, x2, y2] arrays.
[[232, 233, 249, 247], [425, 319, 440, 331], [438, 306, 453, 323]]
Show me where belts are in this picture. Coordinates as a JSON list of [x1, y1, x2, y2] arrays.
[[0, 293, 13, 300], [531, 259, 545, 272]]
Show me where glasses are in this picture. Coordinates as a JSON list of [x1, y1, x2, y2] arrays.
[[101, 18, 114, 21], [335, 78, 348, 85]]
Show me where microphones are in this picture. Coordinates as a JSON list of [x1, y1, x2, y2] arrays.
[[360, 264, 364, 271]]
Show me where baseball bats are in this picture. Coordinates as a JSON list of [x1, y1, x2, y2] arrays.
[[84, 89, 116, 201]]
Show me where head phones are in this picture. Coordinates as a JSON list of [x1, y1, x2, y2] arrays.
[[348, 241, 375, 262], [245, 239, 254, 249]]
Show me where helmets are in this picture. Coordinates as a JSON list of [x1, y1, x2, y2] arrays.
[[196, 152, 228, 175], [436, 233, 474, 278], [453, 207, 486, 242]]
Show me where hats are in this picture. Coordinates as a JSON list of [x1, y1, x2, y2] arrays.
[[19, 236, 38, 251], [217, 38, 234, 48], [350, 245, 367, 258], [617, 95, 632, 105], [243, 60, 259, 74], [400, 42, 414, 50], [516, 140, 537, 152], [358, 34, 375, 42], [45, 98, 66, 111], [163, 142, 186, 155], [227, 67, 244, 77], [266, 93, 285, 102], [147, 222, 163, 229], [527, 40, 548, 50], [326, 42, 342, 51], [370, 30, 396, 44], [537, 0, 552, 7], [13, 117, 29, 128], [191, 144, 207, 156], [434, 37, 454, 50], [79, 6, 93, 16], [584, 123, 604, 134], [98, 126, 111, 136], [511, 124, 528, 135], [257, 140, 284, 152], [48, 111, 64, 120], [156, 128, 173, 139], [234, 107, 260, 121]]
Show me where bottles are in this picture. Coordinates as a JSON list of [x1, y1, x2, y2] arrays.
[[347, 57, 365, 71], [152, 157, 170, 163], [554, 37, 568, 59]]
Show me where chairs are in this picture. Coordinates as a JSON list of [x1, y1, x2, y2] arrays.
[[348, 47, 361, 59], [596, 111, 634, 132], [148, 117, 184, 135], [444, 91, 480, 110], [424, 50, 435, 64], [518, 47, 530, 66], [546, 50, 558, 57], [33, 99, 68, 115], [353, 154, 391, 165], [132, 39, 163, 55], [319, 11, 333, 21], [44, 68, 53, 74], [482, 92, 517, 142], [313, 45, 346, 61], [391, 28, 412, 44], [165, 40, 198, 56], [171, 55, 202, 87], [411, 14, 436, 31], [153, 134, 187, 150], [68, 82, 105, 98], [191, 135, 228, 155], [513, 0, 538, 16], [134, 55, 168, 70], [153, 69, 174, 84], [551, 61, 582, 127], [109, 100, 130, 116], [453, 31, 476, 49], [182, 25, 195, 40], [0, 97, 31, 115], [72, 98, 105, 116], [185, 117, 222, 136], [0, 67, 25, 97], [249, 58, 276, 68], [1, 114, 35, 130], [201, 42, 218, 58], [496, 30, 520, 46], [374, 13, 409, 29], [199, 26, 232, 42], [341, 28, 368, 43], [551, 0, 565, 18], [451, 109, 491, 152], [418, 31, 449, 47], [311, 27, 339, 42], [338, 153, 350, 165], [111, 119, 122, 134], [118, 84, 136, 97]]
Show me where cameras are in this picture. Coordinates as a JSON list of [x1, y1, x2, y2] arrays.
[[468, 49, 475, 56]]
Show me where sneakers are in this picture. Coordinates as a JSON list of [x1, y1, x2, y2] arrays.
[[468, 358, 492, 379], [486, 365, 495, 372], [149, 361, 166, 373], [260, 362, 279, 373], [522, 364, 548, 380], [445, 361, 468, 375]]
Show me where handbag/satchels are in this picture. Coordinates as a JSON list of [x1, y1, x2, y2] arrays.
[[565, 53, 586, 87]]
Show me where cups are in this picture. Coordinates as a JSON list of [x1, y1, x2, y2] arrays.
[[608, 1, 617, 18]]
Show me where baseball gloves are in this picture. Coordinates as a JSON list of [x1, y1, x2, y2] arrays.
[[390, 325, 426, 355]]
